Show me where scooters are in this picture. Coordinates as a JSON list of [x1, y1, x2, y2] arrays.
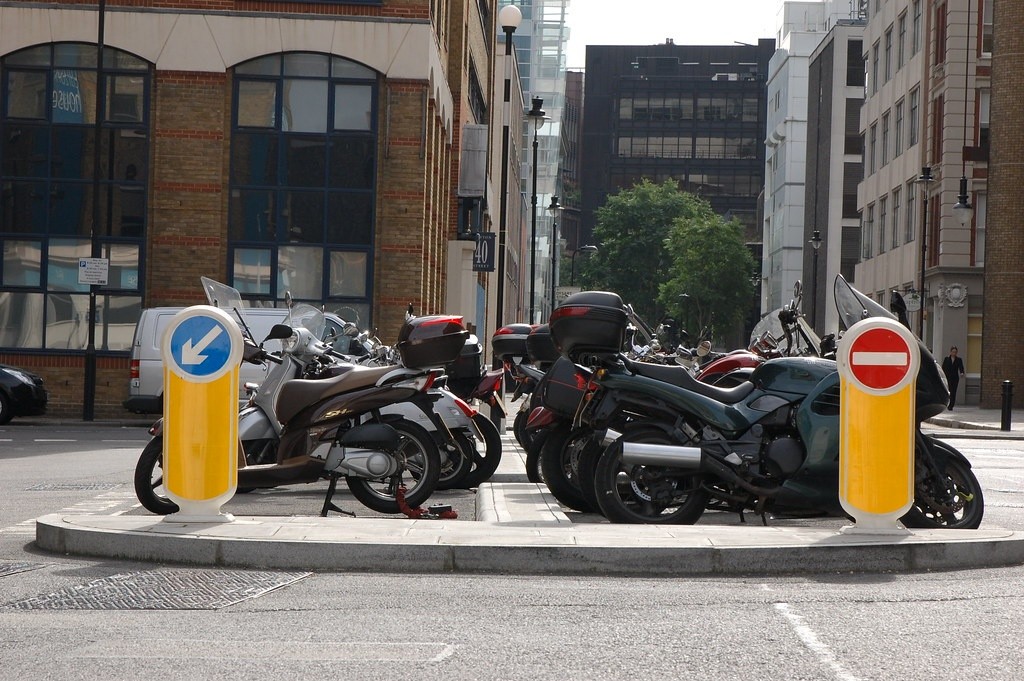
[[133, 274, 470, 518]]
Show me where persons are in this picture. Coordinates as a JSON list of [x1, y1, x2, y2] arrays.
[[942, 346, 964, 411]]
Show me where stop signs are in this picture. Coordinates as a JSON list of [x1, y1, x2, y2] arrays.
[[847, 326, 912, 389]]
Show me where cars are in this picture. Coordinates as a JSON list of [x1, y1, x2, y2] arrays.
[[0, 363, 47, 423]]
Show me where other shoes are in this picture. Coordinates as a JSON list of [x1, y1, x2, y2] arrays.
[[948, 406, 952, 410]]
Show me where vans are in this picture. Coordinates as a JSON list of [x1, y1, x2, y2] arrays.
[[124, 305, 385, 415]]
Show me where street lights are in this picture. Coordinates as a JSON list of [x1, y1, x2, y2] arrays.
[[679, 292, 690, 334], [571, 245, 598, 286], [546, 193, 565, 313], [911, 168, 937, 344], [498, 4, 522, 434], [524, 96, 553, 325], [807, 230, 826, 333]]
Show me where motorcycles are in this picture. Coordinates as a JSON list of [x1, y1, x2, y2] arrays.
[[548, 272, 985, 530], [232, 281, 842, 514]]
